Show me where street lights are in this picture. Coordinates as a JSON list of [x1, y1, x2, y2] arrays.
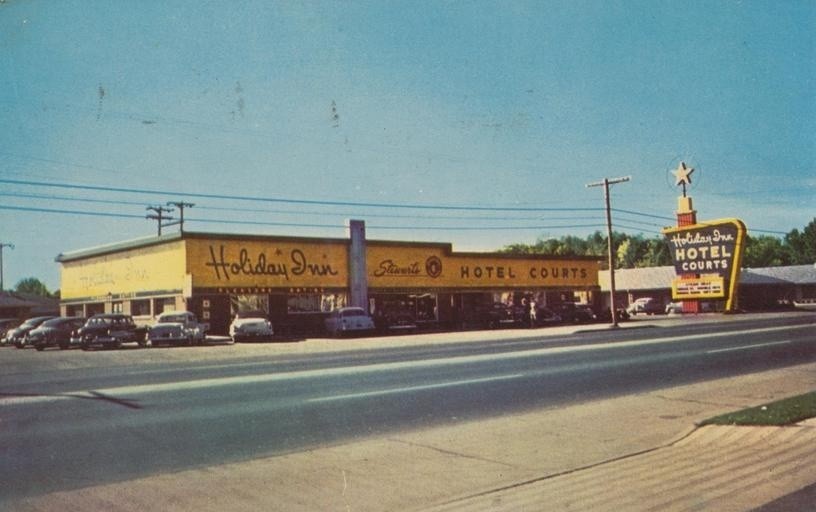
[[0, 242, 15, 298]]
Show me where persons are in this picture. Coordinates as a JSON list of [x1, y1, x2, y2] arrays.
[[528, 297, 540, 329]]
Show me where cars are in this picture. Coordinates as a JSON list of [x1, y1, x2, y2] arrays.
[[763, 285, 816, 307], [0, 309, 207, 351], [227, 310, 275, 343], [453, 291, 714, 326], [323, 306, 377, 336], [375, 302, 417, 331]]
